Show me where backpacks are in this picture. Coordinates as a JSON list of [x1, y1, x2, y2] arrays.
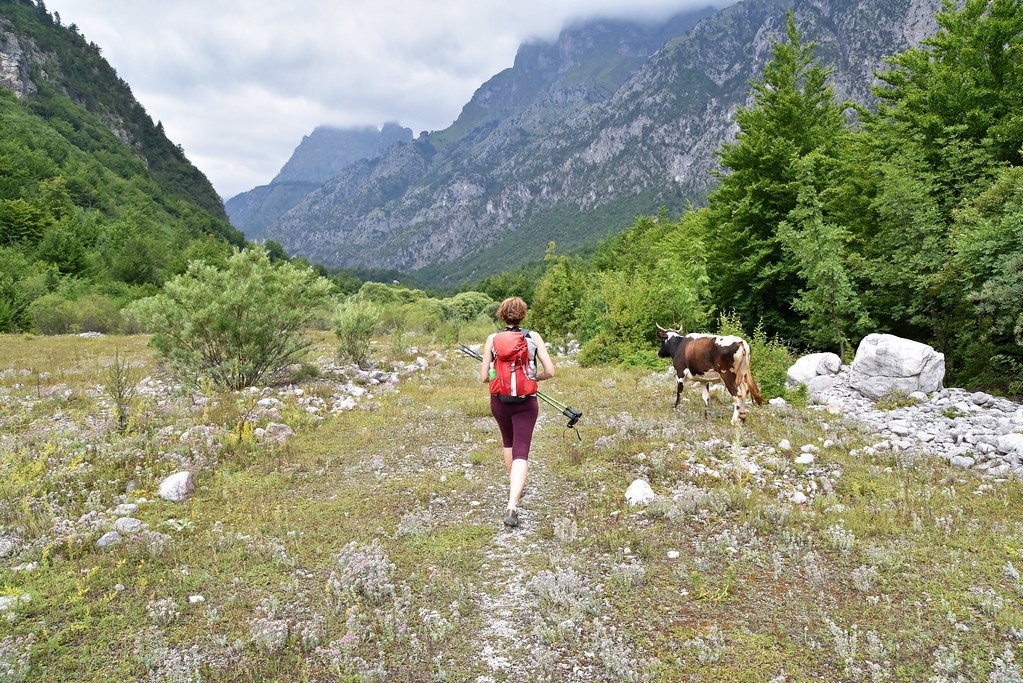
[[488, 328, 537, 403]]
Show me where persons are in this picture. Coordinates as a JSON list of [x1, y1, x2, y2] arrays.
[[481, 296, 555, 528]]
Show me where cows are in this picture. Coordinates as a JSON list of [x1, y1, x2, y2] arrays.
[[656, 322, 764, 426]]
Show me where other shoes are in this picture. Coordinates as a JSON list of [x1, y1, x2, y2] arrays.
[[503, 509, 519, 527]]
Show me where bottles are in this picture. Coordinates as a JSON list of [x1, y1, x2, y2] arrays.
[[527, 361, 537, 380], [488, 362, 497, 382]]
